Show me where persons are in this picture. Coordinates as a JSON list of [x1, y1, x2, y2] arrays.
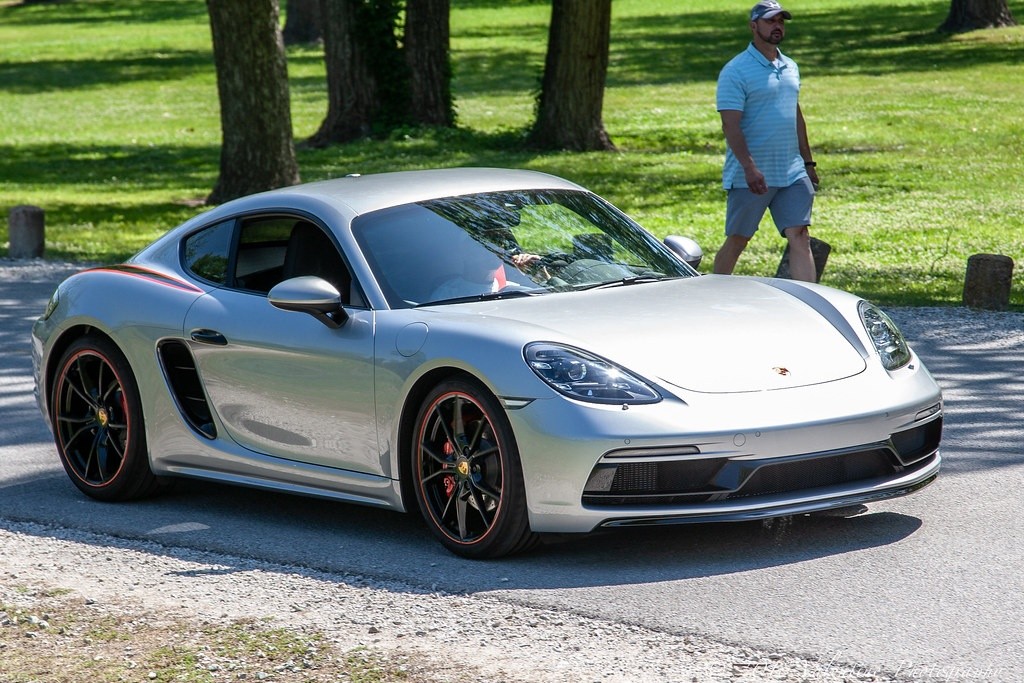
[[429, 221, 552, 302], [711, 0, 824, 283]]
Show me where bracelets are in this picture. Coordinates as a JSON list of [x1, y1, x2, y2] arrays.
[[803, 161, 817, 167]]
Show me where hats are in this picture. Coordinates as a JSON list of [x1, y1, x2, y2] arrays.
[[751, 0, 792, 22]]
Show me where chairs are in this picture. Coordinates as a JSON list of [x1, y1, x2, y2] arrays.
[[283, 224, 348, 303]]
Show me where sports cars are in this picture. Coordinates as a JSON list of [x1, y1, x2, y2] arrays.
[[32, 166, 943, 560]]
